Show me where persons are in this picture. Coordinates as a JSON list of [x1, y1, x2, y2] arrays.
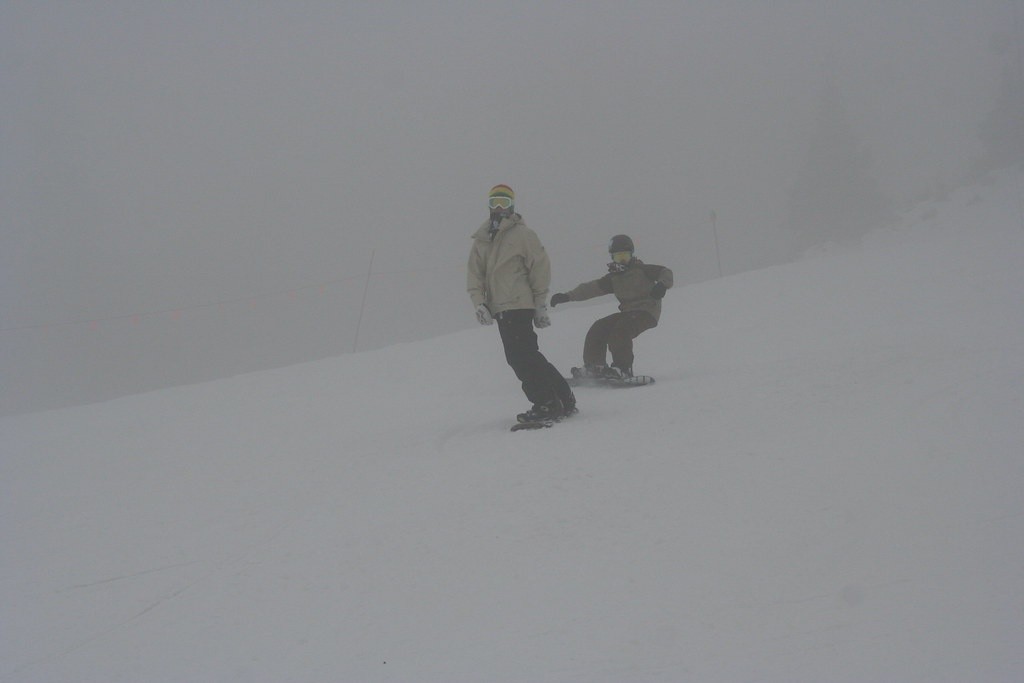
[[550, 235, 674, 379], [466, 184, 576, 421]]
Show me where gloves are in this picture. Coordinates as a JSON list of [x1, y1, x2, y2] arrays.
[[550, 291, 569, 308], [475, 304, 492, 326], [651, 282, 665, 300], [534, 310, 551, 328]]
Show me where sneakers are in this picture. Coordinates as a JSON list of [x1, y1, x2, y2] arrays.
[[517, 396, 576, 422], [571, 363, 633, 379]]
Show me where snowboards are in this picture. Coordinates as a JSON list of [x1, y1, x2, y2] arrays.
[[562, 376, 653, 388], [510, 417, 557, 430]]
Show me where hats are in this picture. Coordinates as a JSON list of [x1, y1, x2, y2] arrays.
[[489, 184, 515, 199]]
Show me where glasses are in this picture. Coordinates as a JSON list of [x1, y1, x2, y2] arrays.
[[488, 197, 511, 210]]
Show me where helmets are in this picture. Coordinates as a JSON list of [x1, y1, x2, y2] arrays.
[[608, 234, 634, 252]]
